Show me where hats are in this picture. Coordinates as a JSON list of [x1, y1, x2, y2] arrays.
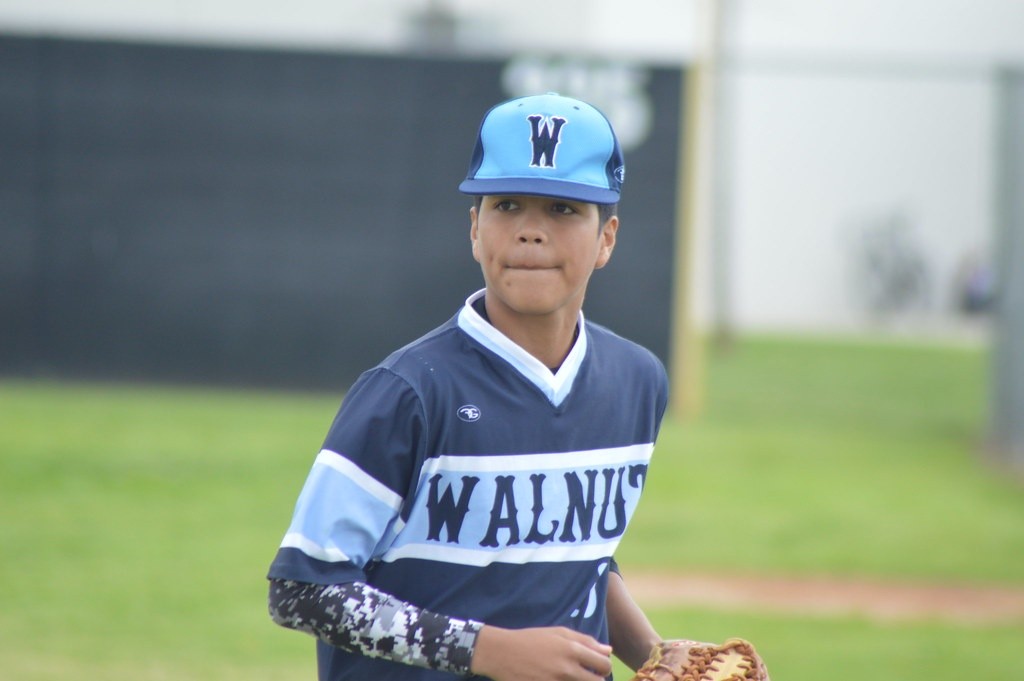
[[459, 92, 626, 207]]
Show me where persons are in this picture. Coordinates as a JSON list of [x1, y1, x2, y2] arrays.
[[267, 93, 772, 681]]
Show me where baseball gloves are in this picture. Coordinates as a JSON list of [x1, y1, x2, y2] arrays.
[[626, 633, 775, 681]]
